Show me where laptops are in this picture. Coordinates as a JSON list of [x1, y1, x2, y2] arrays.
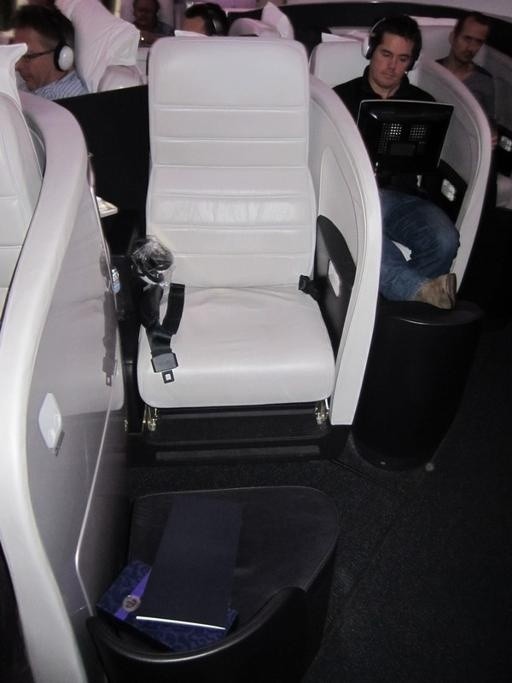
[[357, 99, 455, 187]]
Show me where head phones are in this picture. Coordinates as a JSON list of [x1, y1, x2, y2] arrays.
[[44, 9, 75, 74], [362, 16, 421, 73], [209, 14, 223, 37]]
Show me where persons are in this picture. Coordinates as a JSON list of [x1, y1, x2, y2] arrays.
[[330, 14, 461, 312], [131, 0, 176, 47], [435, 11, 503, 212], [180, 2, 229, 37], [7, 2, 89, 102]]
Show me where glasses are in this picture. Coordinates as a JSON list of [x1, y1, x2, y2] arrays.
[[8, 39, 57, 62]]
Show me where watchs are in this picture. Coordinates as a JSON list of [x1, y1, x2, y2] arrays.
[[141, 32, 145, 42]]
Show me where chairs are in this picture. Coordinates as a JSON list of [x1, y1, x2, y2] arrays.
[[0, 1, 511, 683]]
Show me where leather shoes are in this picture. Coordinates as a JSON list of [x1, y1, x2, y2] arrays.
[[422, 273, 456, 310]]
